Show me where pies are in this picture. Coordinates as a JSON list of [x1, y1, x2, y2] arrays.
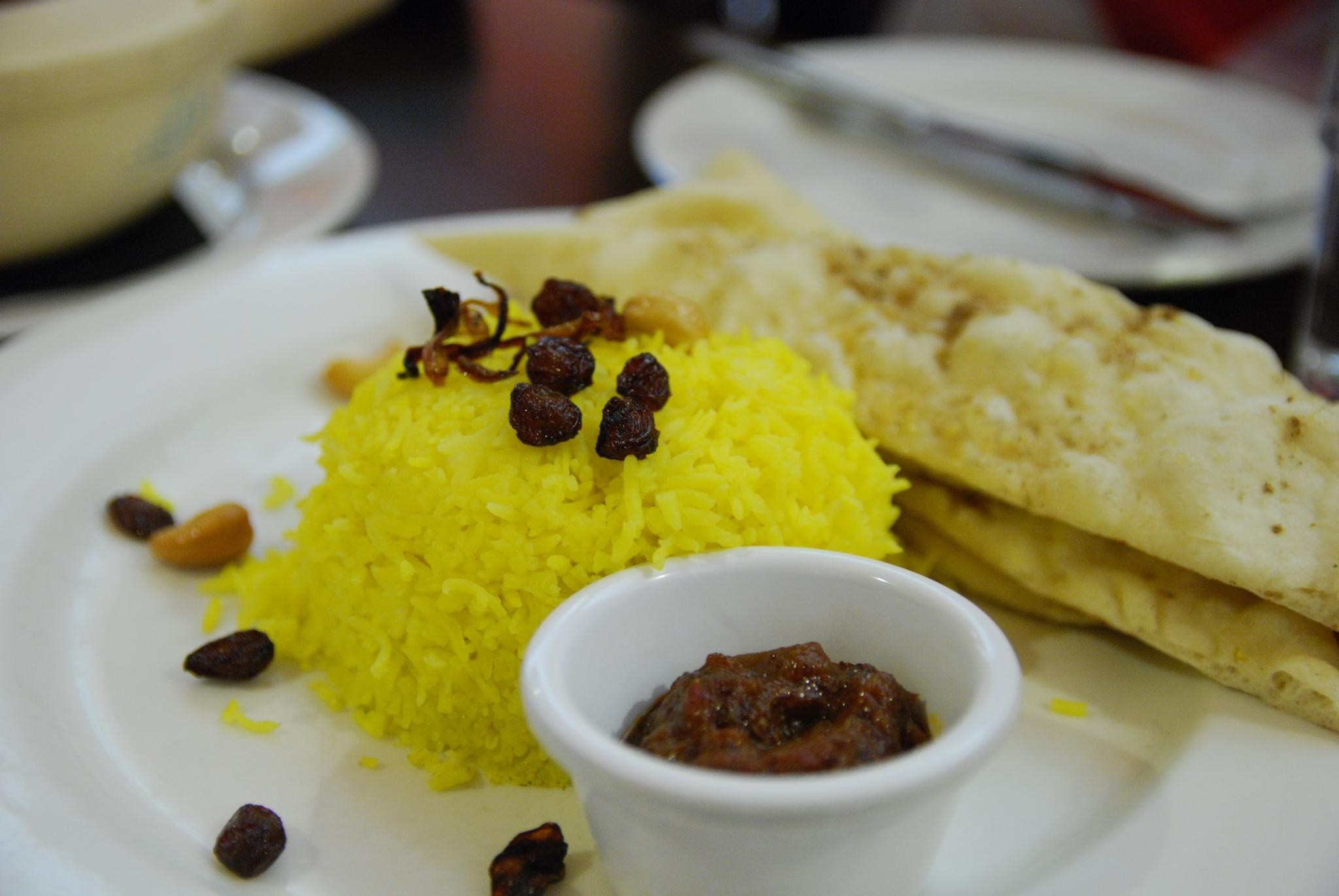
[[430, 148, 1339, 739]]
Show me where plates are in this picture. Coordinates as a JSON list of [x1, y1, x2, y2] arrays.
[[633, 34, 1329, 289], [0, 207, 1339, 896], [0, 68, 384, 338]]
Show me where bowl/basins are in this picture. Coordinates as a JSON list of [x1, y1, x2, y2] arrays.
[[0, 0, 227, 258], [519, 546, 1023, 896]]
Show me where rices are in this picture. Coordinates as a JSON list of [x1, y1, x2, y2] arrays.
[[144, 293, 908, 797]]
